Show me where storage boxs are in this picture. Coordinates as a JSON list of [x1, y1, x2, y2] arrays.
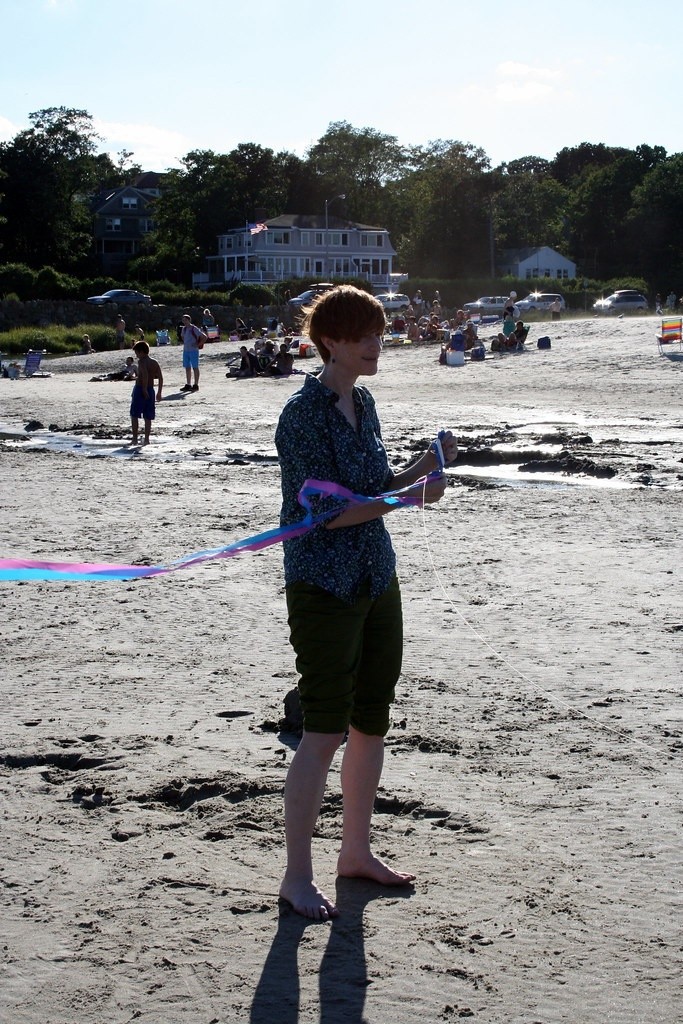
[[446, 349, 464, 365]]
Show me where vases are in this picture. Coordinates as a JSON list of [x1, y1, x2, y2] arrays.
[[7, 367, 18, 377]]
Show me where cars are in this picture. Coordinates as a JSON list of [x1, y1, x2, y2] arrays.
[[86, 289, 152, 309]]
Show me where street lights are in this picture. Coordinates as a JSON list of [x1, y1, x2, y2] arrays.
[[324, 194, 346, 281]]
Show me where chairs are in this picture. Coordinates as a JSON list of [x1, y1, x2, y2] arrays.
[[655, 317, 683, 358], [156, 329, 171, 346], [207, 324, 220, 342], [16, 350, 44, 378], [496, 325, 530, 351]]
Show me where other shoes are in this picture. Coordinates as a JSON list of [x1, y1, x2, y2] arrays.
[[180, 384, 191, 391], [189, 385, 199, 392]]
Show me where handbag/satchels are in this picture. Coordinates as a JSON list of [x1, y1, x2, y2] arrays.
[[299, 344, 317, 358]]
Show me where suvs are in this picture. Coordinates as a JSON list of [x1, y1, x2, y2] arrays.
[[593, 289, 649, 317], [514, 293, 567, 316], [463, 296, 511, 319], [373, 293, 411, 316], [287, 283, 334, 308]]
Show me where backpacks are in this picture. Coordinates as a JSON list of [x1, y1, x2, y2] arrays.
[[184, 325, 203, 349]]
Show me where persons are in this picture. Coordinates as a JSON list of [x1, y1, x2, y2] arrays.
[[129, 341, 162, 445], [655, 291, 676, 311], [93, 357, 138, 381], [204, 288, 526, 377], [82, 333, 92, 355], [115, 314, 126, 351], [179, 315, 208, 391], [275, 284, 457, 924], [549, 298, 561, 321], [132, 324, 145, 340]]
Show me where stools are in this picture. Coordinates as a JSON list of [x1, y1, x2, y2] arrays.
[[436, 329, 450, 342]]
